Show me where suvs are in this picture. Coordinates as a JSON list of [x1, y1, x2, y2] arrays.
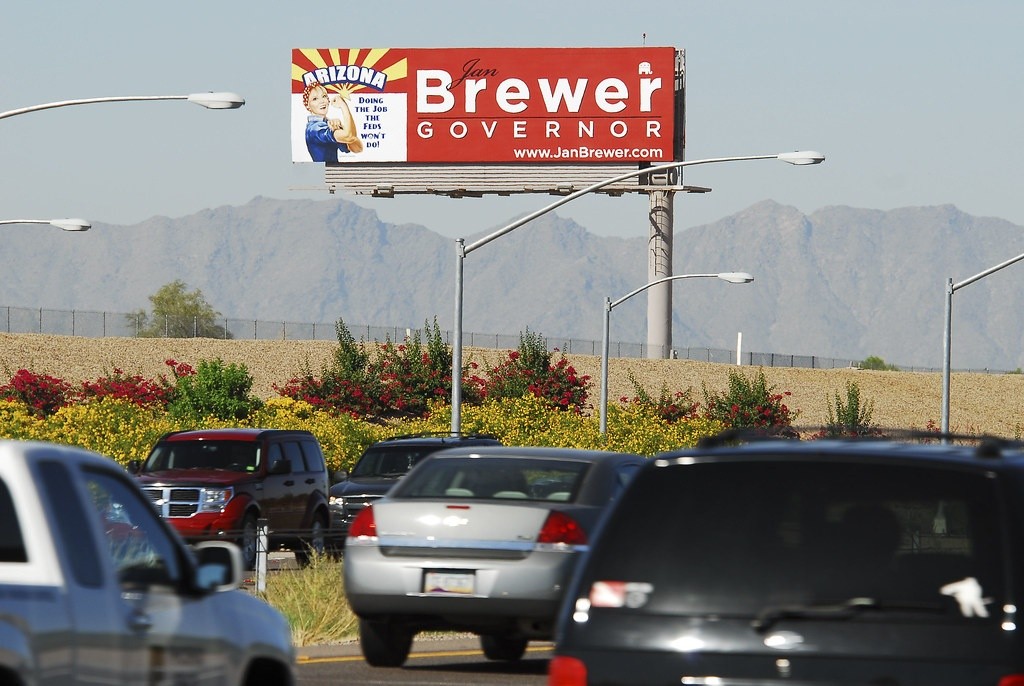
[[0, 437, 299, 685], [544, 430, 1023, 685], [325, 431, 530, 562], [99, 426, 332, 571], [341, 443, 646, 664]]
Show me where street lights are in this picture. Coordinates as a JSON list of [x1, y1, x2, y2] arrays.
[[448, 149, 826, 437], [597, 271, 755, 444]]
